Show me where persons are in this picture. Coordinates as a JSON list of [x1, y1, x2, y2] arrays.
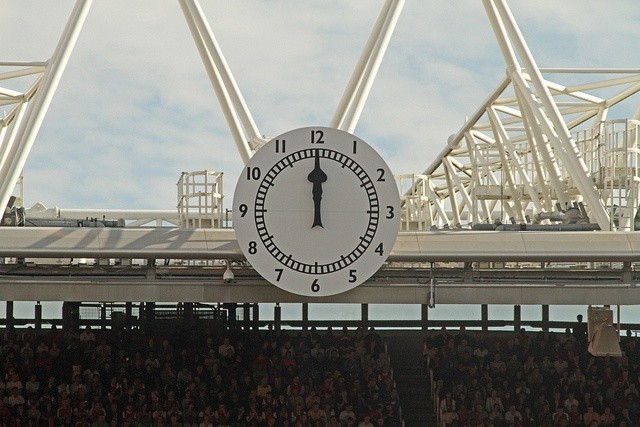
[[1, 326, 403, 426], [425, 314, 640, 426]]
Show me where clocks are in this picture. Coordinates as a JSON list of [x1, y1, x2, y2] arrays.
[[232, 127, 401, 298]]
